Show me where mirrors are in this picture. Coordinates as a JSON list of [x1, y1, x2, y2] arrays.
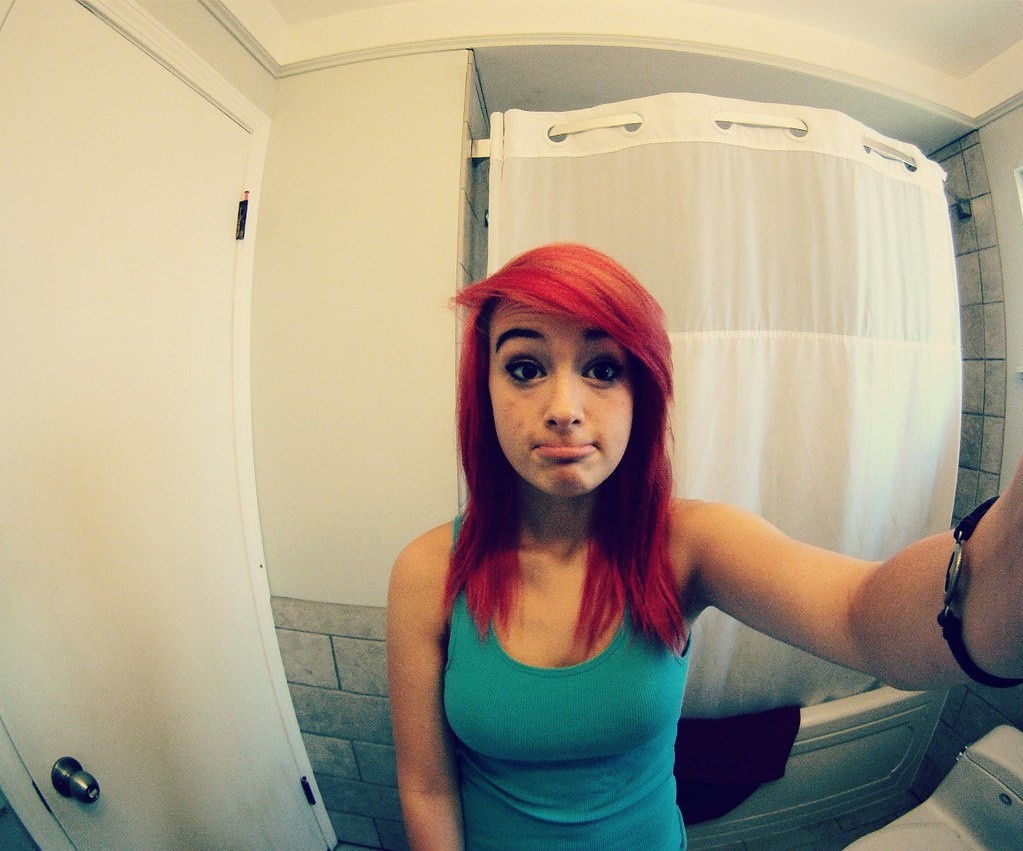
[[0, 0, 1023, 851]]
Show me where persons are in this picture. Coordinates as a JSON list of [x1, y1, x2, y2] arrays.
[[389, 240, 1023, 851]]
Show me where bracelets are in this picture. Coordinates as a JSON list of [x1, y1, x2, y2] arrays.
[[935, 492, 1023, 691]]
